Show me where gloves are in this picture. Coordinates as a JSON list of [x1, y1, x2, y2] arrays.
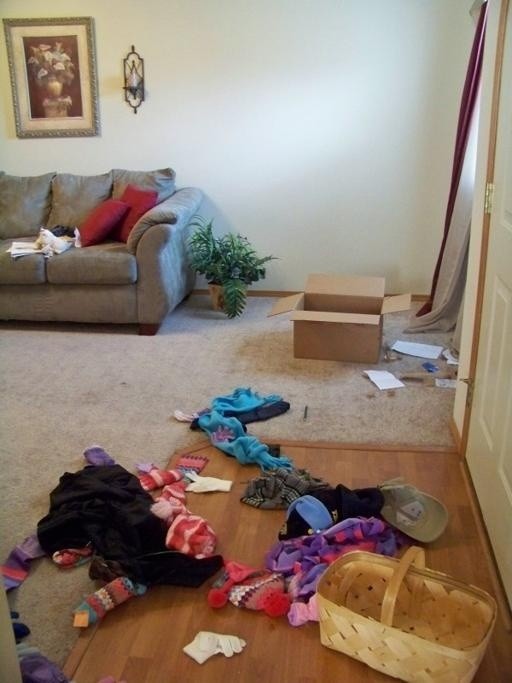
[[136, 463, 234, 508], [180, 630, 247, 666]]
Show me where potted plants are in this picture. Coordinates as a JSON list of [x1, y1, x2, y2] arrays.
[[185, 213, 283, 320]]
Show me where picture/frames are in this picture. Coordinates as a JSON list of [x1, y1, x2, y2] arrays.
[[2, 15, 103, 141]]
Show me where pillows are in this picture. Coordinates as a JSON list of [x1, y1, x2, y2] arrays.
[[0, 166, 176, 248]]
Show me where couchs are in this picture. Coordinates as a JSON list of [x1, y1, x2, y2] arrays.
[[1, 165, 204, 334]]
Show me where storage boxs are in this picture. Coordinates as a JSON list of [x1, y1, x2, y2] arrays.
[[264, 271, 414, 365]]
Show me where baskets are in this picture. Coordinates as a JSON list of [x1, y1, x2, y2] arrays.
[[316, 546, 497, 683]]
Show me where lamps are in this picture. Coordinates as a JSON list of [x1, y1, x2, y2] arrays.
[[122, 45, 145, 115]]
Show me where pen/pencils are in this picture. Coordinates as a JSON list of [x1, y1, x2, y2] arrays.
[[304, 406, 308, 421]]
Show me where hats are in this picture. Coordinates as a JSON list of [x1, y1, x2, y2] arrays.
[[376, 476, 451, 546], [209, 560, 290, 622], [160, 506, 219, 565]]
